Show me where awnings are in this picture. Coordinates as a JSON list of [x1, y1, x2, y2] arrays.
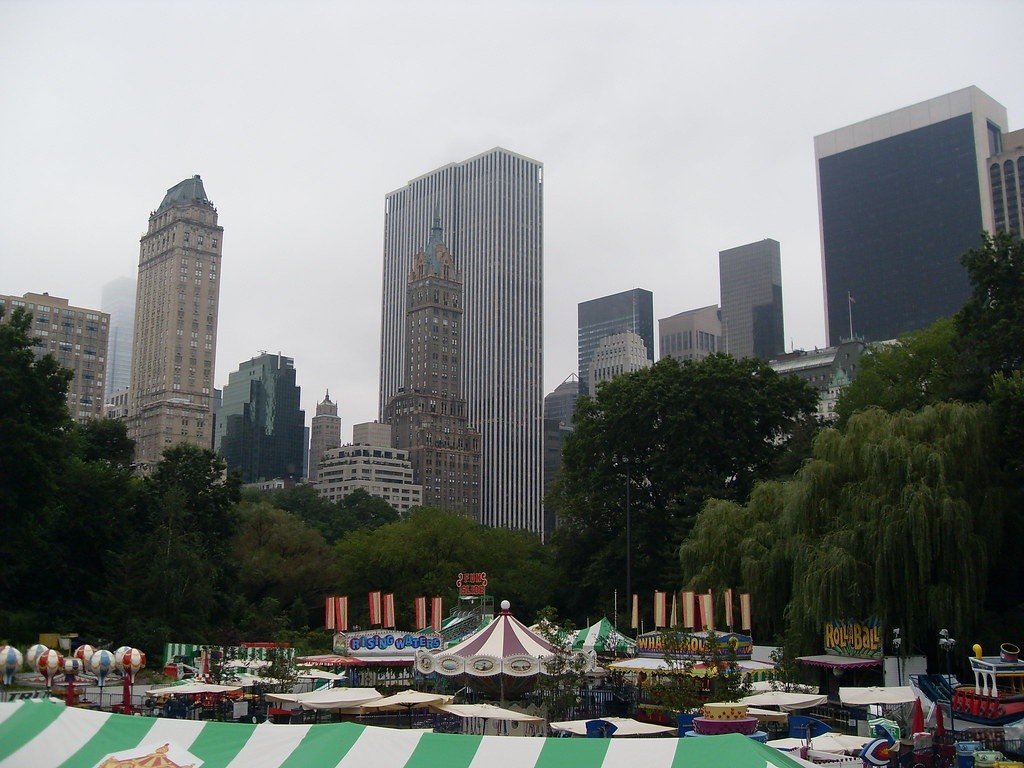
[[292, 654, 415, 668], [795, 654, 883, 668], [608, 657, 784, 679]]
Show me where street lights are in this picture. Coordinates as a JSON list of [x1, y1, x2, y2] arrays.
[[892, 628, 901, 687], [612, 452, 630, 636], [939, 629, 957, 743]]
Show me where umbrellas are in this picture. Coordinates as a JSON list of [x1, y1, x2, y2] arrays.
[[212, 672, 291, 694], [145, 682, 241, 720], [935, 705, 945, 745], [216, 658, 273, 673], [549, 717, 678, 738], [737, 680, 900, 756], [359, 689, 455, 730], [838, 686, 918, 718], [427, 702, 545, 736], [911, 698, 924, 734], [290, 668, 349, 692]]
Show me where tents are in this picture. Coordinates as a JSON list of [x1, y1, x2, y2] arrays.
[[553, 616, 639, 656], [0, 700, 822, 768]]
